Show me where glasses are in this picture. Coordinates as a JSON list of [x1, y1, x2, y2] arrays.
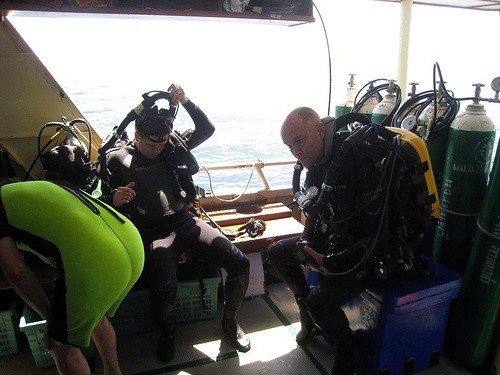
[[145, 132, 169, 143]]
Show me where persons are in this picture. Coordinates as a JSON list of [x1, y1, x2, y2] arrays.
[[0, 180, 145, 375], [267, 106, 421, 375], [105, 85, 251, 362]]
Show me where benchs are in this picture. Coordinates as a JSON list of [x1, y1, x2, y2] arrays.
[[207, 202, 305, 250]]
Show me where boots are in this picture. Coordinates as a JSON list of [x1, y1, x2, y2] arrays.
[[221, 309, 250, 353], [293, 294, 314, 345], [318, 314, 357, 375], [156, 317, 176, 364]]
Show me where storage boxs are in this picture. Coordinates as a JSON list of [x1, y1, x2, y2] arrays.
[[19, 300, 96, 368], [174, 267, 221, 320], [308, 252, 462, 375], [220, 252, 265, 299], [108, 287, 153, 336], [0, 303, 19, 357]]
